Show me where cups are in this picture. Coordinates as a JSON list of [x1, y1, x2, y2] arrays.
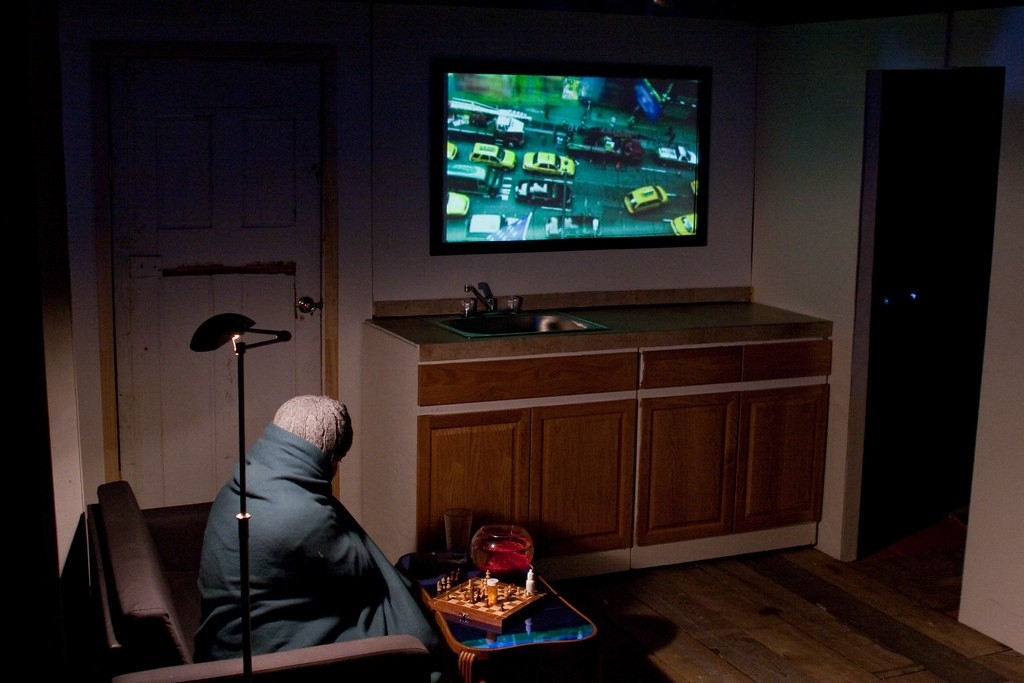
[[443, 507, 472, 551]]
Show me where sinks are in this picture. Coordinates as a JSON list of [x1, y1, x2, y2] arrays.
[[420, 311, 612, 338]]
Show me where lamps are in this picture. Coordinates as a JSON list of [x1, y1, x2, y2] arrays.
[[189, 312, 291, 681]]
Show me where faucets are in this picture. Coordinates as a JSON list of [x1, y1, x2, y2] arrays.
[[464, 283, 498, 312]]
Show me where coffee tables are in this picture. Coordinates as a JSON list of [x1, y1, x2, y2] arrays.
[[395, 547, 599, 682]]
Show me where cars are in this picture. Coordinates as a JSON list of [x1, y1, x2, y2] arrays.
[[464, 215, 523, 240], [446, 192, 470, 217], [624, 184, 668, 216], [513, 180, 574, 207], [566, 131, 642, 164], [671, 212, 696, 236], [469, 142, 516, 171], [546, 214, 602, 238], [522, 152, 575, 179], [448, 142, 457, 160]]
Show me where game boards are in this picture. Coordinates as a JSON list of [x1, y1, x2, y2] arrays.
[[431, 576, 549, 634]]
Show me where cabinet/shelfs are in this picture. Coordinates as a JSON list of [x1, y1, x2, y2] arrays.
[[419, 339, 834, 582]]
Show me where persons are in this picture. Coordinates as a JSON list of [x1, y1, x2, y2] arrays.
[[194, 395, 444, 683]]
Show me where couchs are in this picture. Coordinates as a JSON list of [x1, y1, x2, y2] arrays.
[[82, 480, 436, 683]]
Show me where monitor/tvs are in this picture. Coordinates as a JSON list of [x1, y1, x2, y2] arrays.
[[428, 54, 713, 255]]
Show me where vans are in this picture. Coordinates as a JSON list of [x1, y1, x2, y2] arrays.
[[447, 163, 502, 197]]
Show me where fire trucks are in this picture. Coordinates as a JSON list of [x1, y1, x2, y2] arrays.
[[448, 100, 527, 147]]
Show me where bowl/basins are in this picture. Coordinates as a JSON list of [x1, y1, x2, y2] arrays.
[[469, 523, 535, 577]]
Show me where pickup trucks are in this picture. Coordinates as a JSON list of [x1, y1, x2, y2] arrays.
[[658, 144, 699, 169]]
